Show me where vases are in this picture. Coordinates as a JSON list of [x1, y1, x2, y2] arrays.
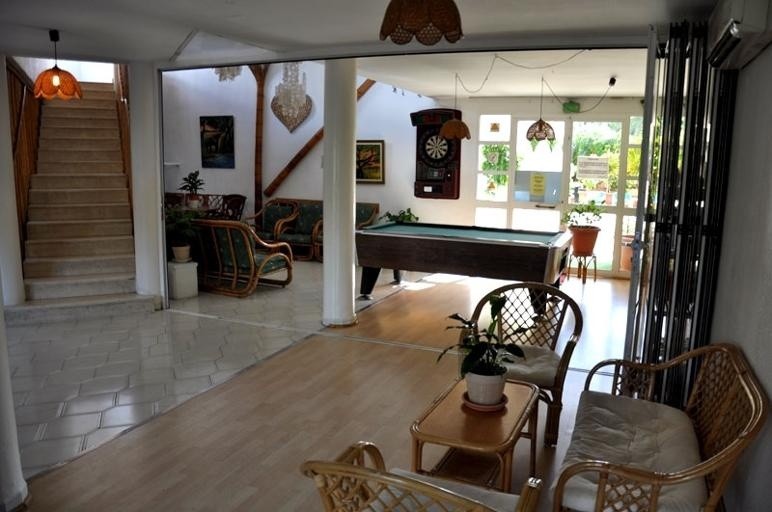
[[171, 243, 194, 263]]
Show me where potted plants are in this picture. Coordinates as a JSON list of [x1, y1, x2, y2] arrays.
[[436, 294, 538, 413], [177, 170, 205, 209], [563, 198, 608, 254]]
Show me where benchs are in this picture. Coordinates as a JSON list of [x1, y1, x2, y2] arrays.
[[551, 342, 771, 511], [176, 218, 293, 298]]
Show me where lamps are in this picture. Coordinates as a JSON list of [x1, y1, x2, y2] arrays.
[[437, 72, 472, 140], [32, 28, 84, 100], [274, 61, 307, 119], [378, 2, 465, 46], [527, 78, 554, 141], [211, 67, 243, 82]]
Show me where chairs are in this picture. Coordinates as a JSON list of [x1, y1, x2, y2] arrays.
[[313, 201, 379, 266], [207, 194, 248, 222], [244, 198, 300, 243], [300, 439, 545, 512], [277, 204, 321, 262], [458, 280, 582, 448]]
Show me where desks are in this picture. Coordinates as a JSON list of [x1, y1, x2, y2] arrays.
[[168, 262, 200, 299], [355, 220, 573, 320]]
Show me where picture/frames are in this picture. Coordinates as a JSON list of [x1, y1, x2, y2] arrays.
[[357, 140, 384, 185], [200, 115, 235, 169]]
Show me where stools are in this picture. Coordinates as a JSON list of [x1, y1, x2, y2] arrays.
[[568, 252, 598, 284]]
